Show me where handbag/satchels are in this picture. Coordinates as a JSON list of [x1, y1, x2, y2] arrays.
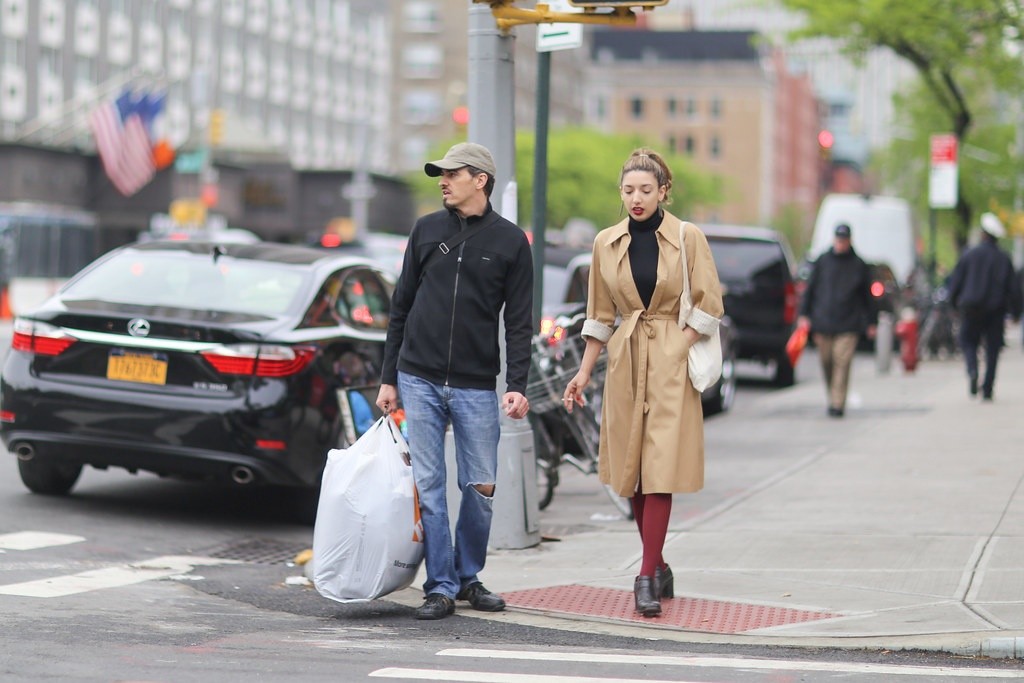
[[310, 413, 426, 604], [784, 325, 809, 369], [677, 221, 724, 392]]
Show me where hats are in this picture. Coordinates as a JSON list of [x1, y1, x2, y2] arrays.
[[423, 142, 498, 180], [979, 212, 1008, 244], [835, 225, 853, 238]]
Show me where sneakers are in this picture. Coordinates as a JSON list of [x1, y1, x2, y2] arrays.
[[415, 591, 457, 620], [458, 581, 506, 613]]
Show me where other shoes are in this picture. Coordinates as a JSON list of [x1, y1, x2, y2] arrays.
[[969, 370, 982, 398], [828, 408, 845, 419], [979, 385, 994, 399]]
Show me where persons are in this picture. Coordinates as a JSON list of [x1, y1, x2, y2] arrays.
[[374, 140, 533, 620], [796, 225, 881, 417], [945, 212, 1024, 400], [563, 147, 724, 615]]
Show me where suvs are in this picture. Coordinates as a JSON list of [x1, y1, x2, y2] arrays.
[[542, 225, 799, 388]]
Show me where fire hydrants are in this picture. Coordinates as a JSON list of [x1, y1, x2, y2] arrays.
[[895, 307, 921, 371]]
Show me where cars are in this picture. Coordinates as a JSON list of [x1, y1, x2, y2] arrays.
[[0, 239, 404, 523]]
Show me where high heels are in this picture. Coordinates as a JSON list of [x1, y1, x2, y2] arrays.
[[633, 575, 663, 615], [650, 563, 675, 599]]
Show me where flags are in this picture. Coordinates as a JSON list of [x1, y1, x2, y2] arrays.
[[96, 80, 171, 198]]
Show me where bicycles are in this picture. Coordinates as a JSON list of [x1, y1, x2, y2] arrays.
[[527, 314, 633, 521]]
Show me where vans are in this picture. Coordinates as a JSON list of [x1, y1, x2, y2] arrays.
[[807, 192, 918, 314]]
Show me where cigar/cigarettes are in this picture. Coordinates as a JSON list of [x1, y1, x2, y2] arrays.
[[561, 398, 573, 401]]
